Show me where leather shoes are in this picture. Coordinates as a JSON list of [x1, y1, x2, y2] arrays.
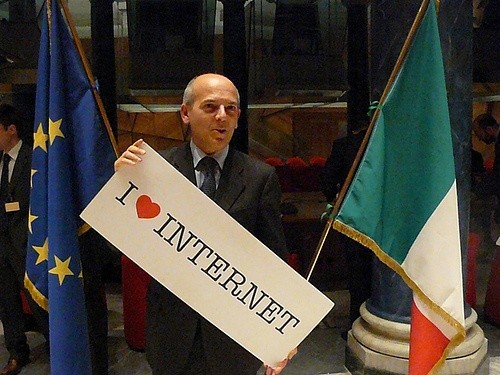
[[0, 355, 29, 375]]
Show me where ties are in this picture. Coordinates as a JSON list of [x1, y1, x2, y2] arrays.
[[0, 152, 11, 194], [198, 157, 217, 199]]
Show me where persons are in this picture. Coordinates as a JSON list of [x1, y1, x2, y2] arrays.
[[113, 73, 298, 375], [319, 106, 376, 341], [470, 113, 500, 225], [0, 104, 32, 375]]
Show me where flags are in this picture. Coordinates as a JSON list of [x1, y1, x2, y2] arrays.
[[23, 0, 118, 375], [331, 1, 467, 375]]
